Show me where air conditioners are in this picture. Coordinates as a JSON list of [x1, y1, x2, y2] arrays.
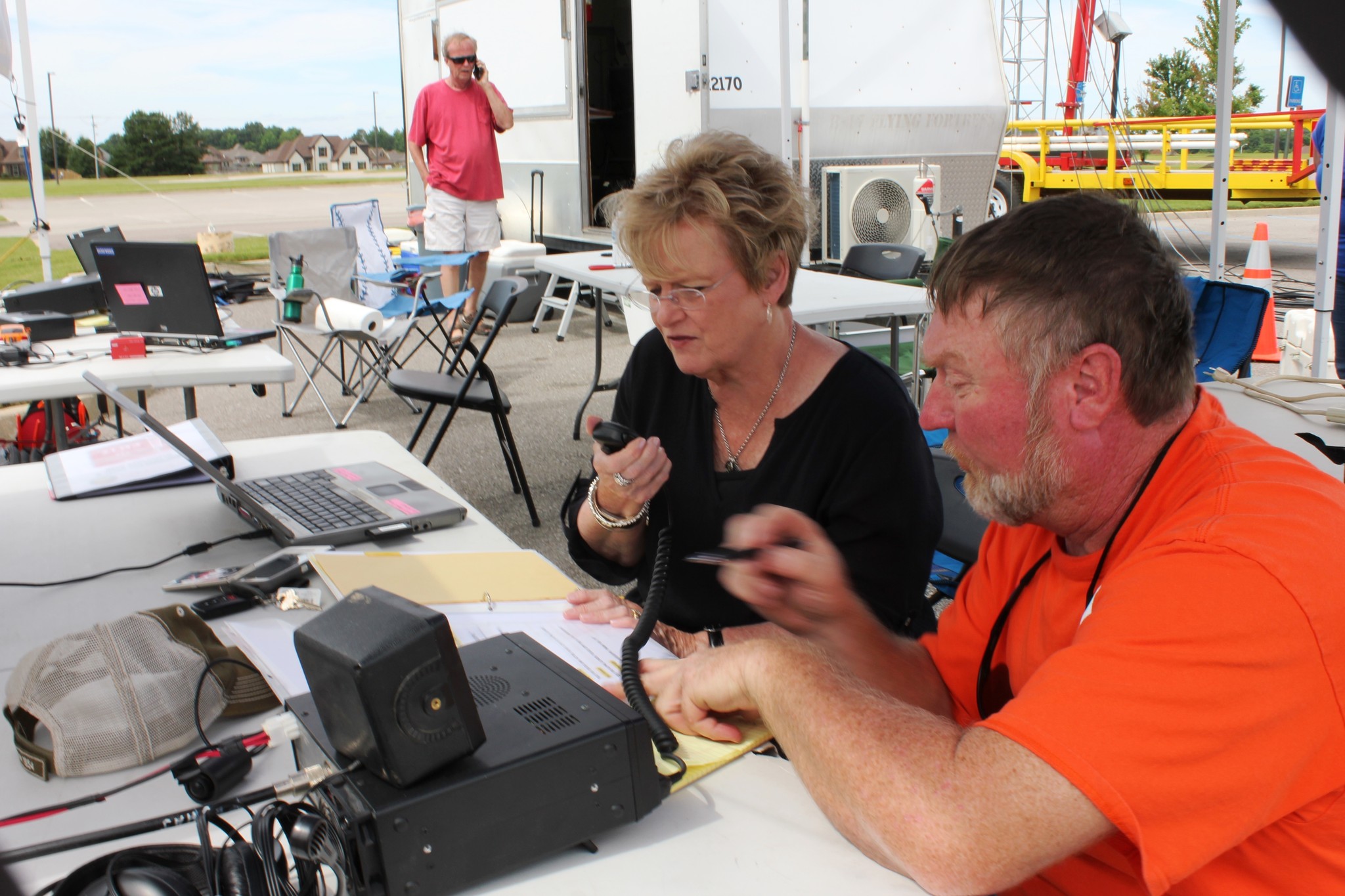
[[821, 166, 943, 265]]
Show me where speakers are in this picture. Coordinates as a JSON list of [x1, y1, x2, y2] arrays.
[[292, 586, 487, 788]]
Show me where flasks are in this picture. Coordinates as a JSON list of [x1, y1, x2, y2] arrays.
[[283, 255, 304, 323]]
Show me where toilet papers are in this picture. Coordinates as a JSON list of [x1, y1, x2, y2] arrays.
[[313, 296, 397, 339]]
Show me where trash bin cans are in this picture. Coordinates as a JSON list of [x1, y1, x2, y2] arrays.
[[405, 204, 468, 299]]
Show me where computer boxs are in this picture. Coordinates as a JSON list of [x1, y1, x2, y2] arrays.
[[0, 272, 107, 316]]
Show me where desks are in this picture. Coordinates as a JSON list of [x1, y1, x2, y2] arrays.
[[0, 428, 927, 896], [535, 251, 935, 439], [1200, 377, 1345, 485], [0, 301, 295, 451]]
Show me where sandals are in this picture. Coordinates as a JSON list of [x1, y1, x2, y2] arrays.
[[460, 308, 493, 335], [448, 325, 464, 348]]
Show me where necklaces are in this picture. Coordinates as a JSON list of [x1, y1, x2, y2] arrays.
[[712, 319, 801, 471]]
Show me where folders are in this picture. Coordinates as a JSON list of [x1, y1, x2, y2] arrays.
[[44, 417, 236, 498]]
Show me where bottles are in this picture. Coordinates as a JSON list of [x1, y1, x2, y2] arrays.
[[611, 211, 632, 267]]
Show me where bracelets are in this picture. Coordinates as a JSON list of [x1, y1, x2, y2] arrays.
[[587, 476, 650, 534], [703, 625, 727, 651]]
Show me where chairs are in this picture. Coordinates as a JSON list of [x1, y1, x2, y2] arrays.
[[266, 198, 541, 529], [1184, 275, 1270, 381]]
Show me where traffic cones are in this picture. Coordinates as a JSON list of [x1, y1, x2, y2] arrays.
[[1238, 222, 1283, 364]]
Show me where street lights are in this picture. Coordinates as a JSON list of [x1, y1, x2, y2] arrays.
[[1093, 8, 1132, 119]]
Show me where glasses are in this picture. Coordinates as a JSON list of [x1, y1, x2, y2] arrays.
[[977, 548, 1052, 723], [447, 52, 477, 65], [625, 257, 744, 311]]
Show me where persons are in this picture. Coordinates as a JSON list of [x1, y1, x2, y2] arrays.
[[558, 130, 944, 660], [409, 32, 514, 347], [636, 191, 1345, 895]]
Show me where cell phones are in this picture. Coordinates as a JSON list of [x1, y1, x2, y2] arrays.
[[474, 63, 481, 80]]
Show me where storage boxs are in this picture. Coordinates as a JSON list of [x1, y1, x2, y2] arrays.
[[384, 227, 415, 257], [479, 238, 552, 323], [400, 241, 421, 295]]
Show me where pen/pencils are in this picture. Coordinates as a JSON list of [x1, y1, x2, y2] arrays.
[[587, 263, 633, 271], [601, 252, 611, 256]]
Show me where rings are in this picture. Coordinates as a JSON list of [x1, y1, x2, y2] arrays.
[[613, 473, 633, 488]]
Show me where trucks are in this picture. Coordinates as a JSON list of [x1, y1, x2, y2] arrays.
[[986, 107, 1324, 223]]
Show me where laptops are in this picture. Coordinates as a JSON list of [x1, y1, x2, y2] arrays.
[[81, 371, 468, 548], [92, 242, 275, 346], [68, 226, 228, 291]]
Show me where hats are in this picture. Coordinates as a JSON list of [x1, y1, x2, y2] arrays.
[[3, 602, 277, 780]]
